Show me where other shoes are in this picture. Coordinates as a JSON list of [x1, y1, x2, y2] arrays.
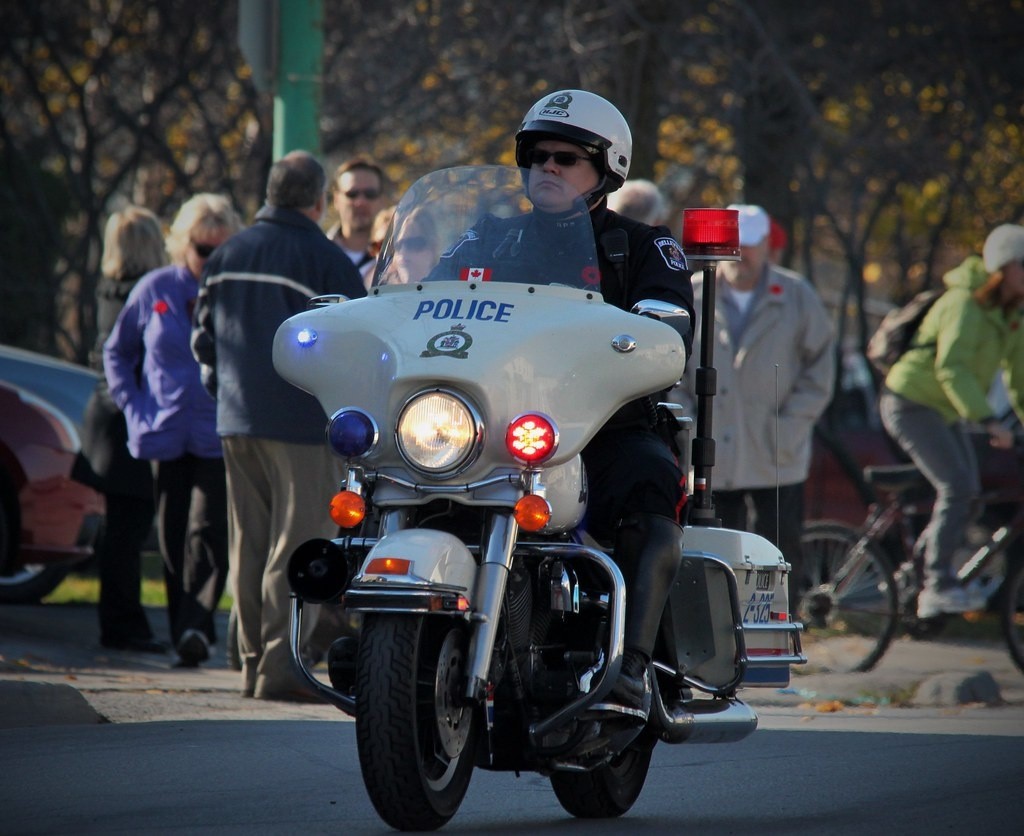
[[254, 682, 331, 704], [171, 651, 200, 670], [177, 628, 211, 663], [102, 638, 171, 655]]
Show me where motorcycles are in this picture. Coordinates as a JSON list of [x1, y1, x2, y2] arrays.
[[271, 164, 810, 833]]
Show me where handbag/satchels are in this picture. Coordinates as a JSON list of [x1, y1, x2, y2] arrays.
[[70, 451, 105, 493]]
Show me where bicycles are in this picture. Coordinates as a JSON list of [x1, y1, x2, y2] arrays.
[[789, 433, 1024, 678]]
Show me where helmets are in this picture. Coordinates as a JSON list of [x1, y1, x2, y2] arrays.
[[516, 89, 633, 193]]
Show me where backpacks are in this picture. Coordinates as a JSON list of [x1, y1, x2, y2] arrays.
[[866, 288, 949, 377]]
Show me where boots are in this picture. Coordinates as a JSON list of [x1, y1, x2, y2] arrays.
[[591, 512, 685, 709]]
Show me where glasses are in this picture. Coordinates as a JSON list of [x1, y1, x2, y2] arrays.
[[527, 148, 593, 165], [339, 189, 382, 199], [190, 239, 214, 257]]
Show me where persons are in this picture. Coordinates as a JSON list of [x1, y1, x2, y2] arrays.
[[79, 144, 385, 706], [881, 223, 1024, 618], [668, 203, 837, 664], [606, 177, 664, 226], [418, 90, 696, 708]]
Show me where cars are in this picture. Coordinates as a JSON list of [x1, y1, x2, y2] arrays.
[[0, 345, 108, 604]]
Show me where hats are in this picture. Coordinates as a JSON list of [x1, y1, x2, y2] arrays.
[[983, 223, 1024, 273], [726, 204, 771, 247]]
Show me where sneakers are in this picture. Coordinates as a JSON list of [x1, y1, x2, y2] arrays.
[[917, 584, 988, 618]]
